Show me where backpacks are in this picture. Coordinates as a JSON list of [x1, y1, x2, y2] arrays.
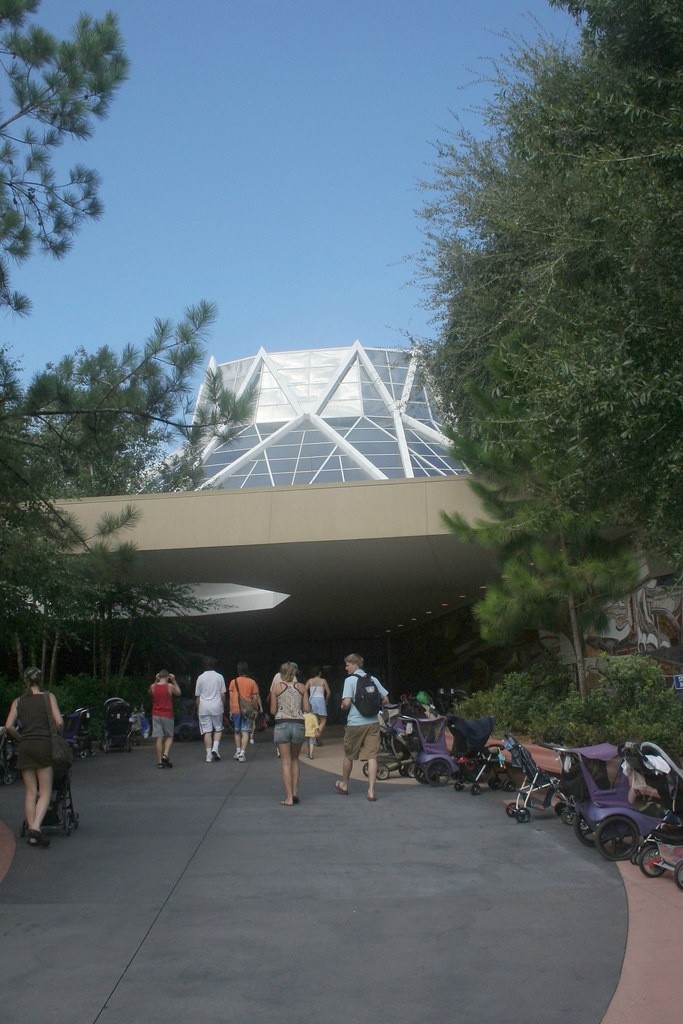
[[352, 673, 381, 716]]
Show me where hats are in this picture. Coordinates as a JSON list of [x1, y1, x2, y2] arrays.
[[23, 667, 41, 686]]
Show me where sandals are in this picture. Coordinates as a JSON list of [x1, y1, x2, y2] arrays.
[[161, 754, 173, 768], [158, 762, 165, 769]]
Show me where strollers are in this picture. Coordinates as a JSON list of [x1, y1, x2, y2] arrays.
[[550, 743, 670, 861], [445, 714, 516, 796], [103, 698, 135, 754], [617, 741, 683, 890], [500, 731, 566, 822], [0, 707, 96, 786], [22, 747, 80, 836], [397, 696, 492, 787], [362, 702, 420, 780], [400, 689, 468, 717]]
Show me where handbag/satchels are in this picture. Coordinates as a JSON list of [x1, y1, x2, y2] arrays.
[[239, 697, 258, 722], [51, 736, 74, 775]]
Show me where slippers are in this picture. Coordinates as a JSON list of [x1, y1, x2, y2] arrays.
[[335, 781, 349, 794], [281, 800, 292, 806], [367, 793, 376, 802], [292, 796, 299, 804]]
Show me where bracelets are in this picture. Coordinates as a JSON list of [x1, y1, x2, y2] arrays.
[[173, 681, 177, 683]]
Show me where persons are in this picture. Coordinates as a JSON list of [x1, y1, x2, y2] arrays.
[[195, 656, 226, 763], [5, 665, 65, 847], [334, 653, 389, 801], [228, 661, 330, 762], [141, 669, 182, 770], [271, 662, 312, 806]]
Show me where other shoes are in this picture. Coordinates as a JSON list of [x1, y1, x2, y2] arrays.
[[307, 752, 314, 759], [239, 754, 247, 762], [234, 751, 240, 759], [250, 738, 254, 744], [212, 748, 220, 760], [316, 735, 322, 747], [206, 755, 213, 762], [28, 827, 51, 846]]
